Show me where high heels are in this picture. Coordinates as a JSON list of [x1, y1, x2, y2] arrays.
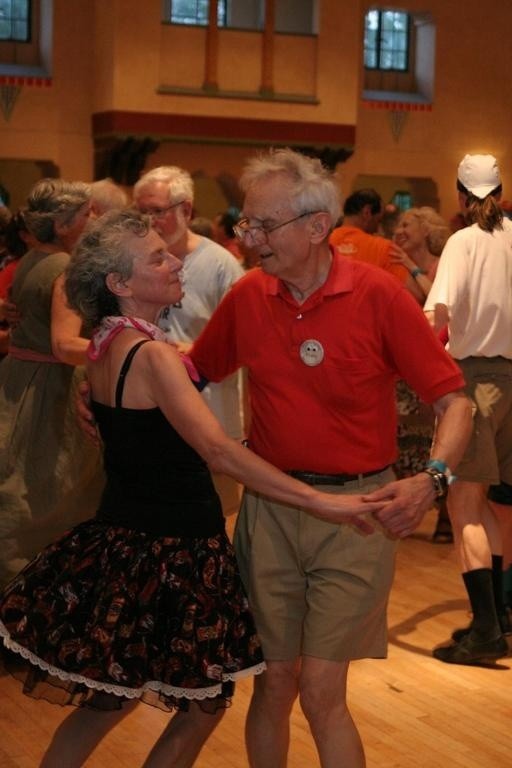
[[432, 518, 452, 543]]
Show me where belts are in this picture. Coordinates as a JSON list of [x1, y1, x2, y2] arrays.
[[288, 470, 378, 486]]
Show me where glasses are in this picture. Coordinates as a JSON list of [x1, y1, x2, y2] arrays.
[[231, 213, 311, 242], [141, 202, 182, 218]]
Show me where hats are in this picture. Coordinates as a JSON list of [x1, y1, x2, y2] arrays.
[[457, 153, 502, 200]]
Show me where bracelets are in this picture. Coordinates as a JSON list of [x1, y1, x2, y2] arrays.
[[424, 460, 457, 486]]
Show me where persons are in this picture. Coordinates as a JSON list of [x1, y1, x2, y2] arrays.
[[1, 210, 395, 768], [73, 145, 474, 767], [1, 156, 511, 663]]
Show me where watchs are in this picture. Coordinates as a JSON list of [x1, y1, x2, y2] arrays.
[[424, 469, 448, 508]]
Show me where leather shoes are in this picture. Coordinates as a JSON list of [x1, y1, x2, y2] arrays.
[[434, 636, 508, 661], [452, 612, 512, 642]]
